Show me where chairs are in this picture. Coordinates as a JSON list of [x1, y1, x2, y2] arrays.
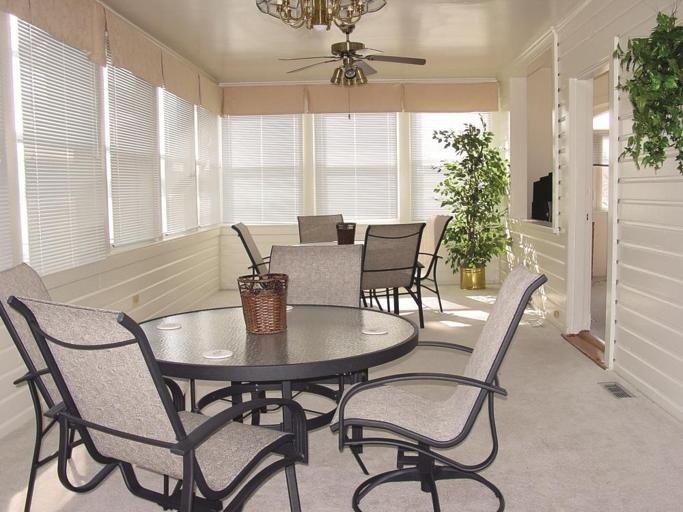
[[385, 214, 453, 313], [0, 261, 169, 512], [229, 221, 270, 292], [7, 295, 310, 512], [296, 212, 368, 306], [258, 241, 365, 454], [328, 265, 549, 511], [360, 221, 425, 330]]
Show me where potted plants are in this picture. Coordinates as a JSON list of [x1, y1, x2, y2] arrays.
[[431, 110, 517, 290]]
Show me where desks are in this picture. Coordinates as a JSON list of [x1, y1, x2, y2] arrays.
[[329, 239, 370, 246]]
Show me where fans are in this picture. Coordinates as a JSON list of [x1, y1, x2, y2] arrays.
[[277, 22, 427, 74]]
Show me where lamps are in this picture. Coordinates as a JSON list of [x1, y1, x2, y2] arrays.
[[330, 57, 367, 119], [256, 1, 387, 31]]
